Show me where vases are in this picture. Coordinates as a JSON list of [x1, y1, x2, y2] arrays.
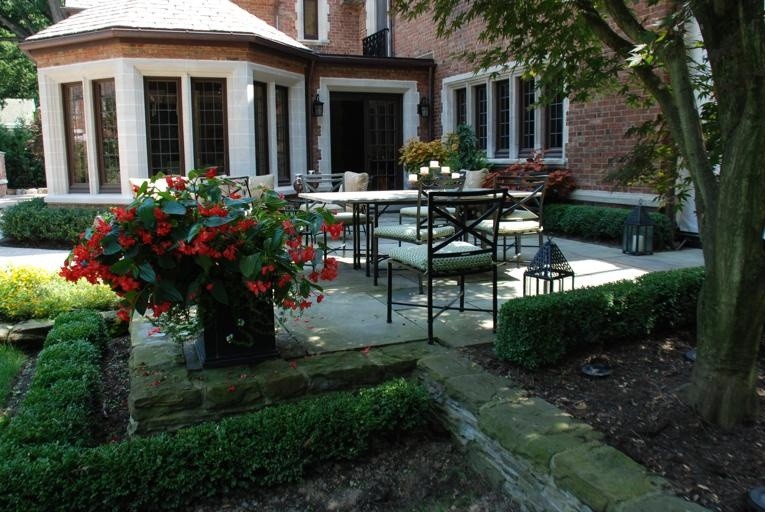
[[191, 279, 286, 367]]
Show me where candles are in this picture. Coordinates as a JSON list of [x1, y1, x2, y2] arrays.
[[452, 172, 460, 179], [440, 166, 450, 174], [407, 173, 417, 182], [429, 161, 438, 169], [420, 165, 428, 175]]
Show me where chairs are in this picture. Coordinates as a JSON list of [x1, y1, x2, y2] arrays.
[[370, 175, 469, 295], [327, 168, 373, 259], [293, 184, 352, 249], [381, 186, 508, 348], [295, 168, 375, 270], [467, 169, 551, 271], [399, 170, 489, 248]]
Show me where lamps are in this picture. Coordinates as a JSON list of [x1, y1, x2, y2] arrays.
[[417, 95, 429, 117], [312, 93, 322, 117], [524, 231, 577, 298], [622, 198, 657, 257]]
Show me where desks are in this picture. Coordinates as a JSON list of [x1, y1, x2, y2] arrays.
[[300, 182, 545, 287]]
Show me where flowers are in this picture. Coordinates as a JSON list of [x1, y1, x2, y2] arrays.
[[57, 167, 341, 333]]
